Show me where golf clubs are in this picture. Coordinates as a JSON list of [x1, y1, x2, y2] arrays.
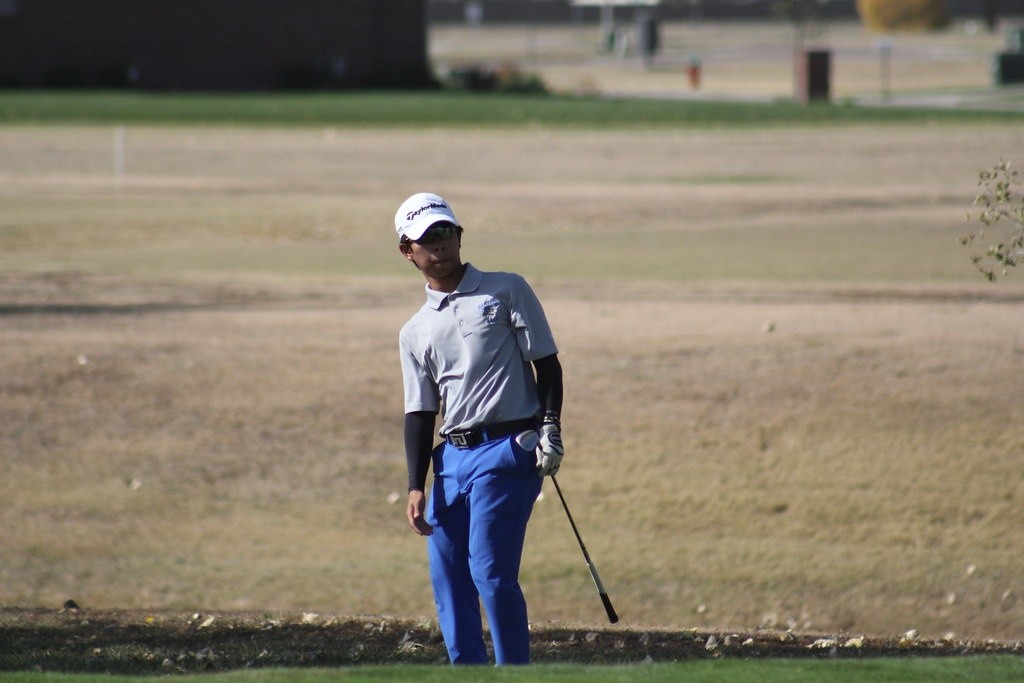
[[514, 429, 620, 624]]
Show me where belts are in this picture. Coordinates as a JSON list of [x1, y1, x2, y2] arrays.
[[448, 417, 537, 450]]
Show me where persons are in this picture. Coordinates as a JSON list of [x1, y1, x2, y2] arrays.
[[393, 193, 565, 668]]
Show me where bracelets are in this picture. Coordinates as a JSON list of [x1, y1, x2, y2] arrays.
[[544, 410, 560, 419]]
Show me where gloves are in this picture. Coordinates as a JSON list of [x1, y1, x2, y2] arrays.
[[535, 416, 564, 477]]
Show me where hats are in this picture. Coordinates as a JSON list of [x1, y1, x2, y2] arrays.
[[395, 192, 459, 242]]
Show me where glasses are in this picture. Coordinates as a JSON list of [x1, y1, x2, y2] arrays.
[[407, 222, 457, 245]]
